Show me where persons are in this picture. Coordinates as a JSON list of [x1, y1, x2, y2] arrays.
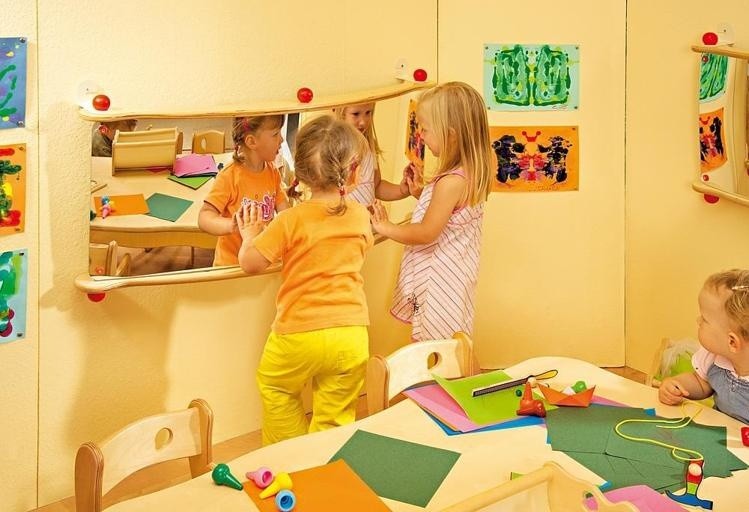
[[198, 114, 290, 268], [370, 82, 497, 387], [92, 119, 137, 156], [335, 103, 414, 213], [658, 269, 749, 425], [235, 115, 374, 447]]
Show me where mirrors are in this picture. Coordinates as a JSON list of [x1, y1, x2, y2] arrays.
[[75, 61, 436, 304]]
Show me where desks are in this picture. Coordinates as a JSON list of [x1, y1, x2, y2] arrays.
[[87, 153, 229, 253], [105, 356, 749, 512]]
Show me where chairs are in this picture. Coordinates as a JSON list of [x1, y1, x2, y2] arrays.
[[364, 331, 477, 417], [189, 127, 226, 154], [75, 397, 218, 512]]
